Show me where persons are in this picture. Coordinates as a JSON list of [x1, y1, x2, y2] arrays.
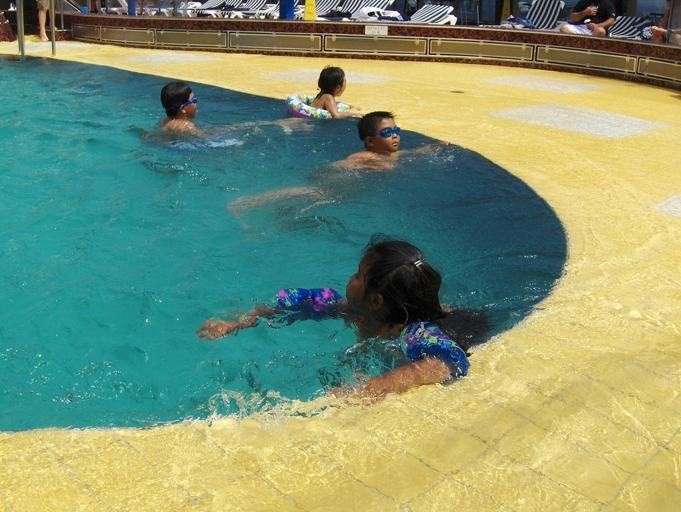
[[224, 108, 450, 222], [36, 0, 60, 43], [648, 1, 681, 49], [0, 11, 18, 44], [231, 64, 367, 137], [196, 232, 499, 411], [557, 0, 618, 40], [140, 82, 226, 151]]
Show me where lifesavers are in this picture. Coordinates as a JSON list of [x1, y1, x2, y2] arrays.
[[286, 94, 352, 119]]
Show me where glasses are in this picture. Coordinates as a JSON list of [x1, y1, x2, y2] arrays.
[[182, 96, 198, 109], [375, 126, 401, 138]]
